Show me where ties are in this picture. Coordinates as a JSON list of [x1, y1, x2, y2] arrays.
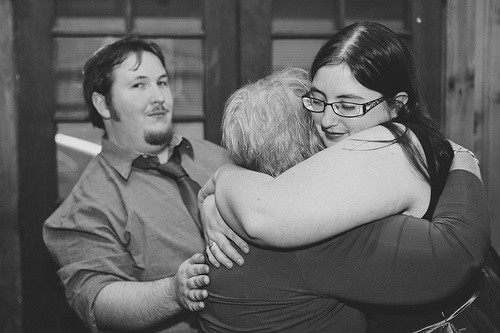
[[132, 149, 203, 234]]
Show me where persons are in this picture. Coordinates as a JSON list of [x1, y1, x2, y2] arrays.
[[43, 34, 233, 333], [194, 64, 499, 333], [196, 22, 454, 269]]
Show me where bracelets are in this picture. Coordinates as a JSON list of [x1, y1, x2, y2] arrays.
[[453, 146, 481, 165]]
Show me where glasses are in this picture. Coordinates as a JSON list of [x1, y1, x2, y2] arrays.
[[301, 91, 387, 118]]
